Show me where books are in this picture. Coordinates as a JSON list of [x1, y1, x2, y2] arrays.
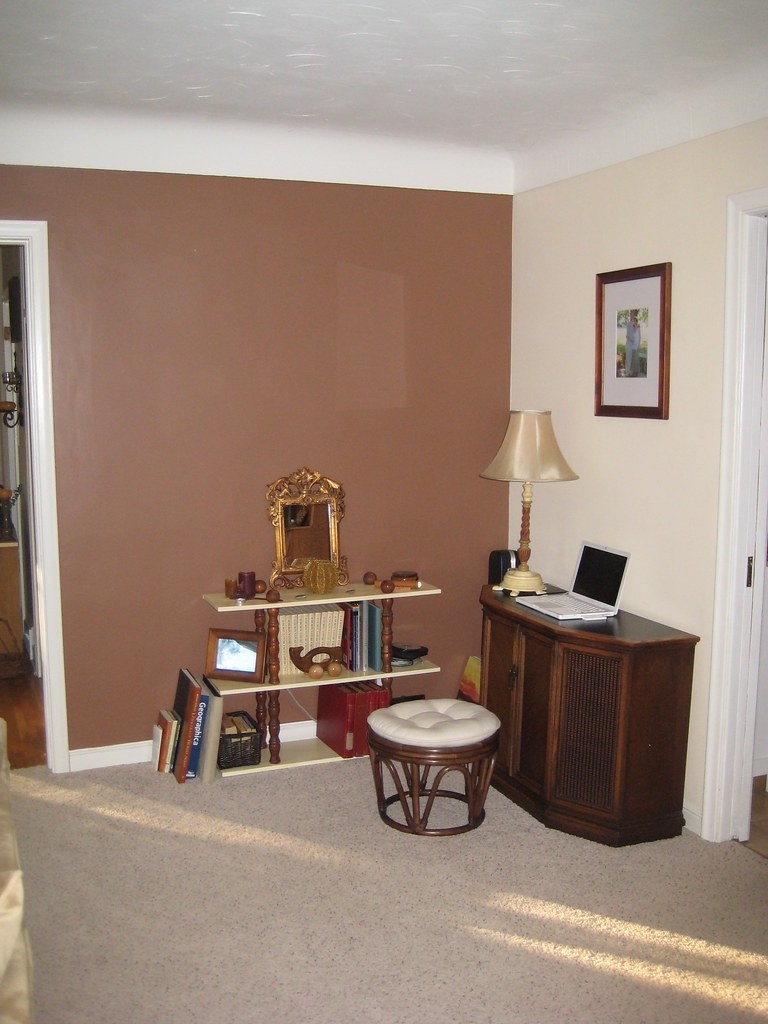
[[151, 669, 223, 784], [264, 600, 383, 677], [316, 656, 481, 759]]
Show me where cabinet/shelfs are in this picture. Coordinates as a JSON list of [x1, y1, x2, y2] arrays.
[[479, 583, 701, 848], [203, 580, 442, 779]]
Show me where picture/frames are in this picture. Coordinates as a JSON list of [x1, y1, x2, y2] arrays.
[[205, 627, 267, 684], [283, 504, 314, 532], [594, 261, 672, 420], [265, 466, 350, 592]]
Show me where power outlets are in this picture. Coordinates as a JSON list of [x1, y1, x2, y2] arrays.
[[24, 621, 34, 660]]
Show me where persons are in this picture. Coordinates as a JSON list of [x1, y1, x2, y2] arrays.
[[625, 310, 641, 377]]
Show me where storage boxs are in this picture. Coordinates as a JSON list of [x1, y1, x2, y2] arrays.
[[217, 711, 267, 770]]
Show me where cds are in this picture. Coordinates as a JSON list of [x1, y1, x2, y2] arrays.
[[392, 642, 421, 650]]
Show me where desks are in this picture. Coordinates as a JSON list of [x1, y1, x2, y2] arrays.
[[0, 524, 23, 654]]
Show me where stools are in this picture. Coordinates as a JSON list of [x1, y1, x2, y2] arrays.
[[366, 697, 501, 836]]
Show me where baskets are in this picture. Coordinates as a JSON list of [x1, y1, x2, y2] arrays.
[[217, 710, 264, 770]]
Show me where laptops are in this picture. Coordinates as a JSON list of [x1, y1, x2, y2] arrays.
[[516, 541, 631, 620]]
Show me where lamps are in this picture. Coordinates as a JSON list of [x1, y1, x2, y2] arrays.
[[479, 410, 579, 596]]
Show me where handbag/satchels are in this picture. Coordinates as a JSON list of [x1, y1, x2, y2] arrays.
[[0, 618, 33, 680]]
